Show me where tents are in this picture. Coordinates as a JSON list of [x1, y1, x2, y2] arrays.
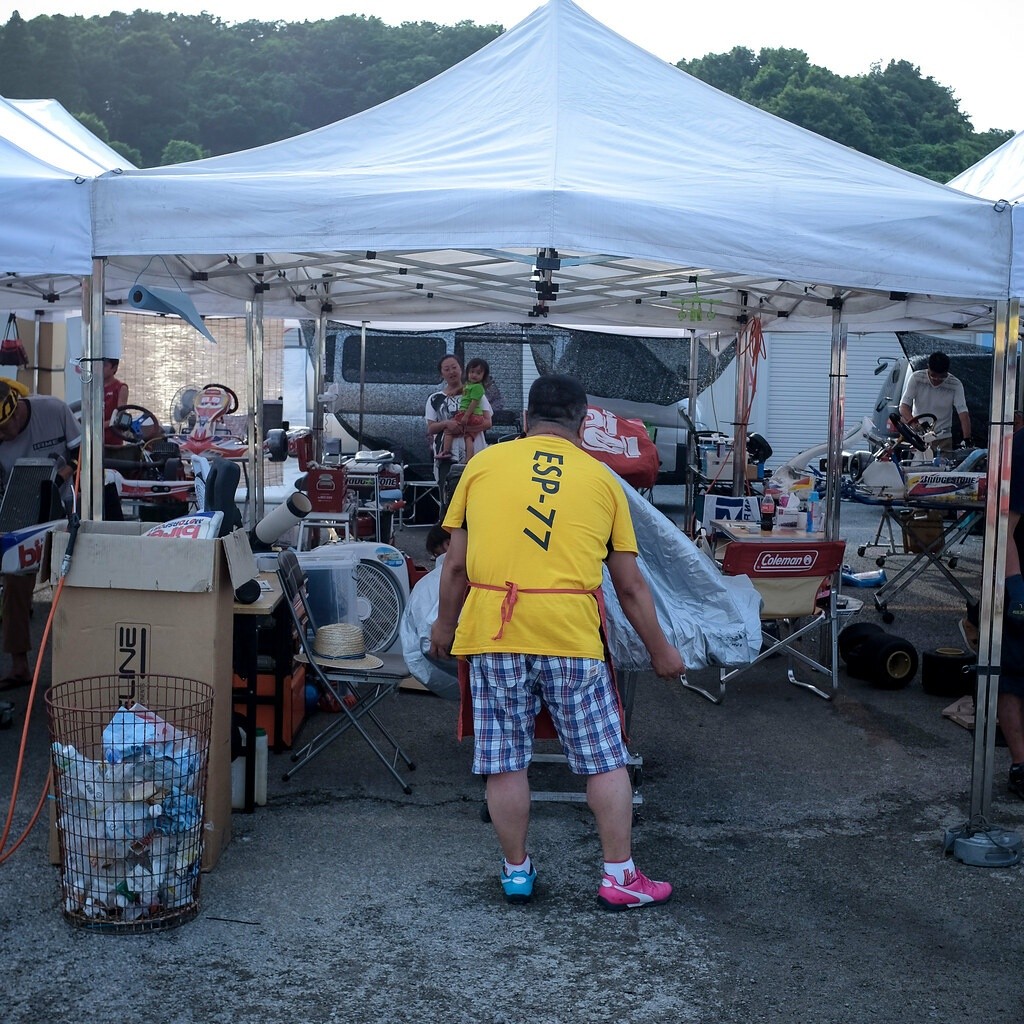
[[837, 133, 1023, 840], [86, 0, 1012, 872], [0, 96, 257, 540]]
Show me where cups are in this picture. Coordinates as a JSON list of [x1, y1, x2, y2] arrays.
[[798, 512, 808, 530]]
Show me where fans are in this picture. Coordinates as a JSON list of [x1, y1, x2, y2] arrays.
[[307, 541, 410, 688]]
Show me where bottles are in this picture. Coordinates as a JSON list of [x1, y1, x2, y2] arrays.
[[806, 491, 820, 533], [762, 489, 774, 531]]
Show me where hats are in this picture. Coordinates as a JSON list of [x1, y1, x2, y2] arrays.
[[293, 622, 383, 670], [0, 378, 28, 424]]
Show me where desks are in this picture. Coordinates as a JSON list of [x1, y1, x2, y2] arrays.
[[861, 498, 988, 623], [691, 463, 772, 536], [231, 569, 306, 813], [706, 518, 828, 667]]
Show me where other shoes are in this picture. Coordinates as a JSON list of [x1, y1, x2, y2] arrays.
[[434, 451, 451, 459], [0, 673, 32, 690], [1007, 763, 1024, 788]]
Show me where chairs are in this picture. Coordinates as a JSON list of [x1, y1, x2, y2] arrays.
[[275, 548, 415, 795], [647, 444, 689, 533], [394, 455, 445, 528], [679, 538, 846, 705], [348, 473, 386, 543]]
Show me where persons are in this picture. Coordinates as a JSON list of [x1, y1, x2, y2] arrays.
[[434, 358, 489, 464], [0, 377, 82, 689], [997, 410, 1024, 797], [898, 352, 971, 469], [428, 372, 686, 910], [425, 355, 494, 523], [102, 358, 128, 447]]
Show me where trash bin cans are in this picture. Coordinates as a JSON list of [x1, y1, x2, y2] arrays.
[[41, 669, 219, 937]]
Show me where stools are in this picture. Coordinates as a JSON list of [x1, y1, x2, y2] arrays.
[[296, 511, 351, 552]]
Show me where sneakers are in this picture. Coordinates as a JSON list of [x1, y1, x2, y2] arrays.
[[500, 861, 537, 900], [597, 865, 673, 911], [958, 618, 979, 655]]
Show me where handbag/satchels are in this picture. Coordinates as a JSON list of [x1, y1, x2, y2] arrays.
[[0, 313, 29, 365]]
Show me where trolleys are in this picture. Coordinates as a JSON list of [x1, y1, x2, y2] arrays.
[[476, 465, 653, 829]]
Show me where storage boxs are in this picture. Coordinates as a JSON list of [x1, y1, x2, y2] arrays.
[[698, 443, 749, 481], [32, 517, 258, 870], [255, 550, 360, 636], [903, 472, 987, 499], [307, 463, 346, 513]]
